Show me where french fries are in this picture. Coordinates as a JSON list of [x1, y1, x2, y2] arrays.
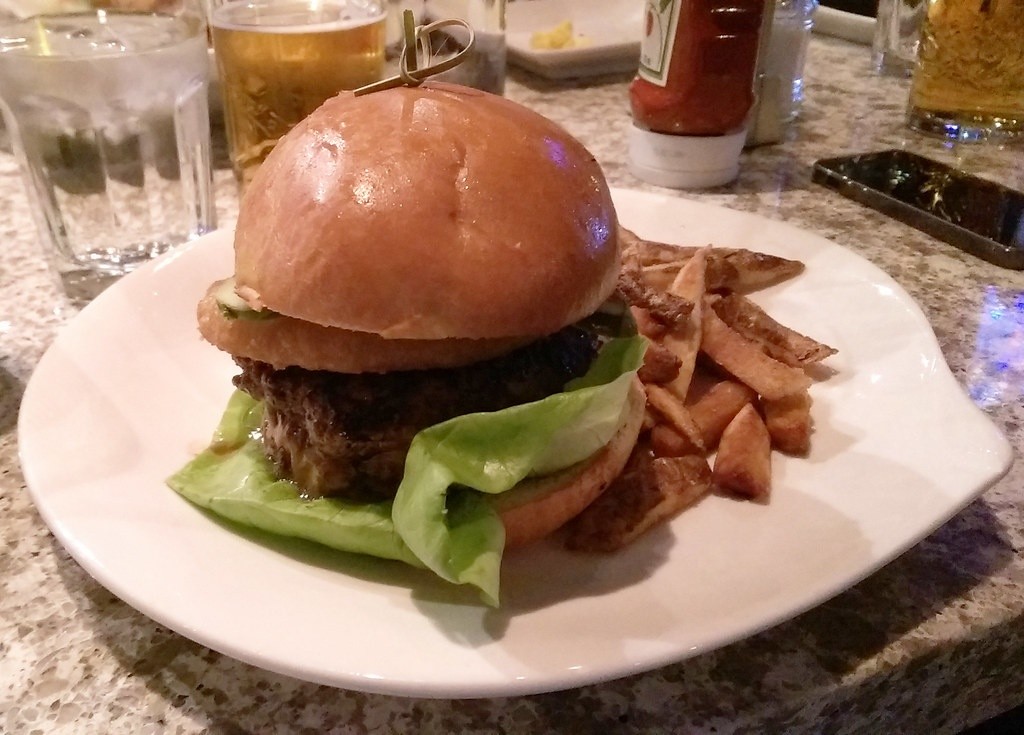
[[561, 229, 840, 552]]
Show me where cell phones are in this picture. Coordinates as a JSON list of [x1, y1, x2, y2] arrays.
[[811, 148, 1024, 271]]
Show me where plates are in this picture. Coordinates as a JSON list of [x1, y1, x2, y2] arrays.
[[505, 0, 646, 79], [19, 190, 1014, 698]]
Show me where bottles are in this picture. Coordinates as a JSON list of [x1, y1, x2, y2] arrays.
[[747, 0, 819, 143]]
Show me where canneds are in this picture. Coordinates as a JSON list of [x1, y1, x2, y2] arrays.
[[623, 0, 765, 189]]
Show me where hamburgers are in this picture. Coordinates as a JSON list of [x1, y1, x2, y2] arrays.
[[164, 80, 653, 611]]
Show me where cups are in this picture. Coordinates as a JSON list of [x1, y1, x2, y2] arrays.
[[872, 0, 929, 77], [906, 1, 1024, 145], [209, 0, 387, 203], [424, 0, 507, 96], [0, 0, 217, 302]]
